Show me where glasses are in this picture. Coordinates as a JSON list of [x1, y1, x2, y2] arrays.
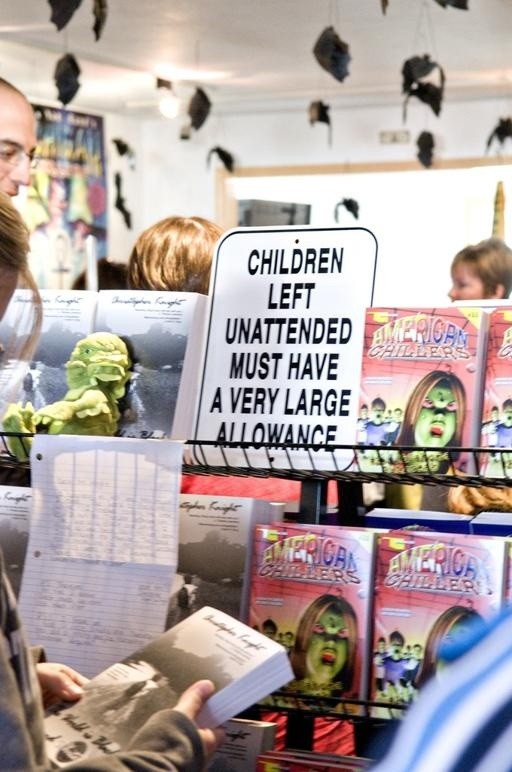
[[1, 148, 41, 169]]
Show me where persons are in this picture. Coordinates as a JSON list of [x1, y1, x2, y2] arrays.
[[0, 76, 40, 198], [448, 238, 512, 302], [128, 217, 228, 294], [0, 187, 224, 772]]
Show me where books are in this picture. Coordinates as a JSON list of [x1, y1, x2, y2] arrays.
[[250, 523, 390, 715], [0, 487, 44, 592], [90, 290, 208, 467], [24, 604, 296, 765], [166, 494, 286, 635], [0, 287, 96, 453], [480, 297, 512, 477], [354, 305, 486, 476], [370, 533, 512, 721]]
